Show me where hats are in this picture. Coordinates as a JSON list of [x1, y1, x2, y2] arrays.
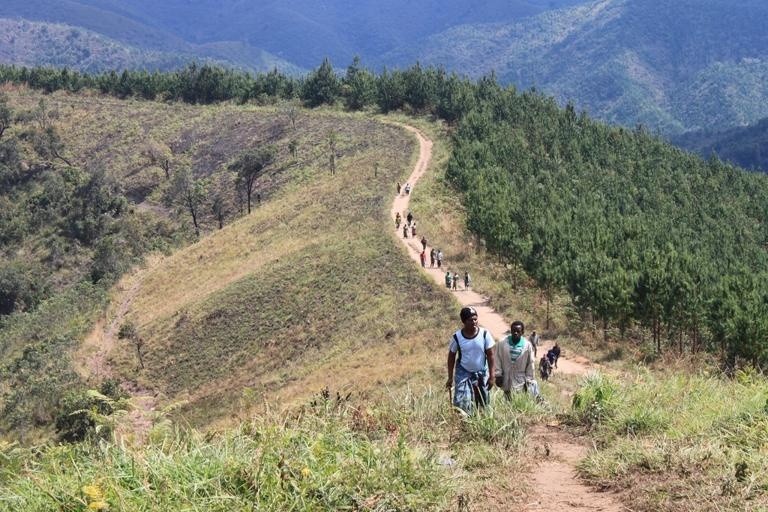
[[460, 307, 477, 323]]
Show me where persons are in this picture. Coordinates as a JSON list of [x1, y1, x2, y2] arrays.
[[538, 342, 561, 381], [419, 236, 447, 269], [397, 182, 411, 196], [445, 271, 471, 291], [529, 330, 539, 356], [395, 212, 416, 238], [446, 307, 496, 413], [495, 320, 535, 401]]
